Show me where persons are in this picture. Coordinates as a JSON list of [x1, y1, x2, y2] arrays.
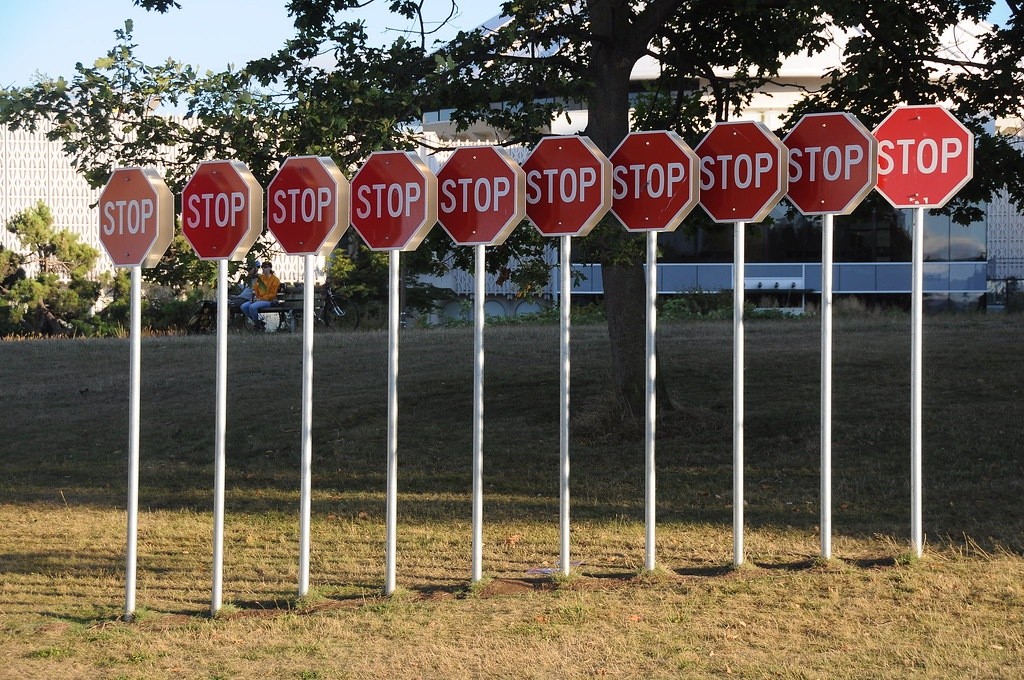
[[241, 260, 280, 335], [228, 261, 260, 312]]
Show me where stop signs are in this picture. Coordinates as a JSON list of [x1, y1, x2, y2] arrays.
[[780, 112, 870, 215], [690, 123, 777, 225], [182, 162, 250, 261], [434, 147, 514, 246], [95, 167, 159, 270], [520, 137, 603, 234], [609, 134, 692, 231], [266, 156, 339, 254], [869, 104, 975, 209], [348, 151, 424, 250]]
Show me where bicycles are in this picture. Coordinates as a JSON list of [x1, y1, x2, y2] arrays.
[[294, 275, 361, 330]]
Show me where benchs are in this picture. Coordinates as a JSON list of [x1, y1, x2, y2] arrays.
[[228, 288, 328, 337]]
